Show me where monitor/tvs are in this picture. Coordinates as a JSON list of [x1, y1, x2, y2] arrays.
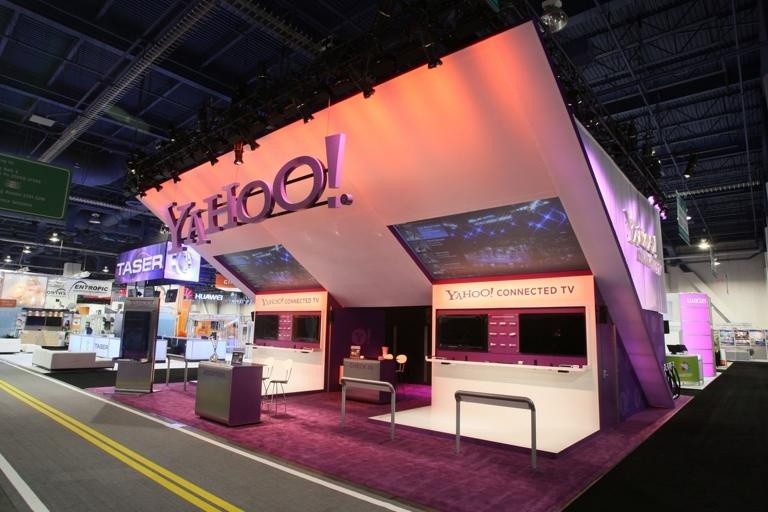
[[128, 289, 136, 297], [165, 289, 178, 302], [144, 287, 154, 297]]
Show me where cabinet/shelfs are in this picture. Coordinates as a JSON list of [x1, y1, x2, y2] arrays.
[[163, 336, 226, 392]]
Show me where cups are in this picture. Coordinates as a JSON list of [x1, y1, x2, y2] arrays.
[[382, 346, 389, 358]]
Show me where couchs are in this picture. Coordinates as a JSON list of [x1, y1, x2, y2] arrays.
[[31, 347, 114, 370]]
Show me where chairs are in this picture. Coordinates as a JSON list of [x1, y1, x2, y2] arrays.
[[256, 358, 291, 420], [384, 354, 408, 395]]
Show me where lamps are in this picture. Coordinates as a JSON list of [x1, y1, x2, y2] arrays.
[[205, 151, 219, 166], [354, 76, 375, 98], [136, 187, 146, 199], [3, 205, 101, 262], [244, 131, 261, 150], [152, 181, 162, 191], [422, 41, 443, 69], [232, 142, 244, 165], [296, 101, 314, 124], [170, 169, 181, 184]]
